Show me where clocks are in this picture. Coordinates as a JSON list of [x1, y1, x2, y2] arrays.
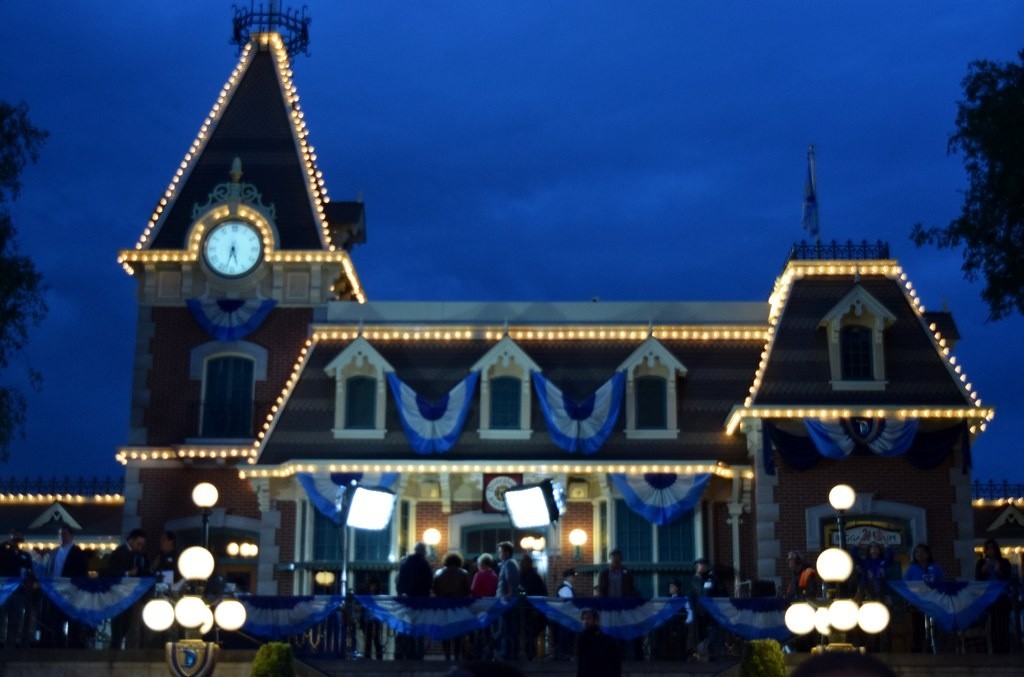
[[202, 216, 266, 280]]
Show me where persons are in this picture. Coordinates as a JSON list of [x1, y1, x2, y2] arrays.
[[359, 578, 386, 659], [0, 522, 31, 647], [975, 537, 1013, 653], [498, 541, 520, 660], [668, 580, 683, 662], [432, 550, 471, 660], [687, 558, 722, 642], [462, 551, 549, 659], [904, 544, 944, 654], [105, 530, 160, 651], [472, 553, 498, 661], [151, 530, 182, 649], [395, 542, 433, 660], [787, 554, 818, 597], [599, 549, 637, 597], [41, 523, 87, 649], [556, 567, 578, 598], [577, 610, 621, 677], [850, 530, 893, 652]]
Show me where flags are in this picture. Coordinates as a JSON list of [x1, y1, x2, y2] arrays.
[[802, 148, 818, 236]]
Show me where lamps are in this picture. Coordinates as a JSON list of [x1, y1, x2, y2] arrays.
[[140, 482, 246, 635], [423, 528, 441, 559], [518, 533, 547, 552], [569, 528, 587, 560], [784, 484, 890, 636]]
